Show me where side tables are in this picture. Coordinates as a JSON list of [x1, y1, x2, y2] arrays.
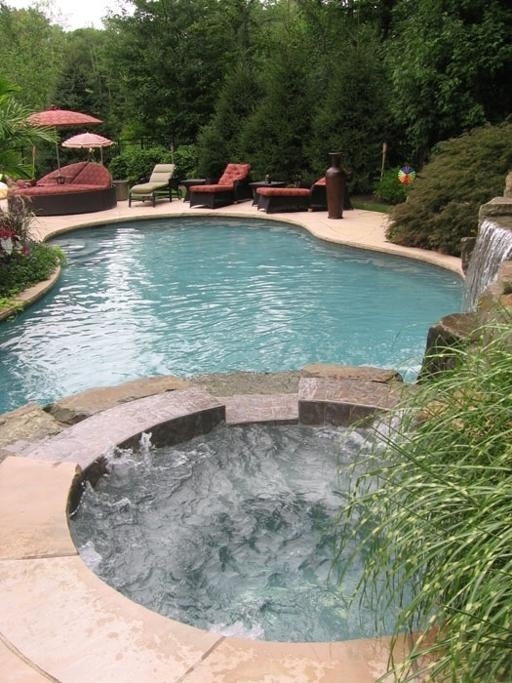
[[180, 179, 206, 202], [248, 181, 287, 198]]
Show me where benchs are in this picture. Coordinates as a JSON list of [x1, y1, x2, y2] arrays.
[[251, 187, 312, 214]]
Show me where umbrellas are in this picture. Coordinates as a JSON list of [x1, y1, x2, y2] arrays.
[[13, 103, 105, 175], [61, 131, 114, 160]]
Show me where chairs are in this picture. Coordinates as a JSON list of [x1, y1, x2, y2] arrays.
[[311, 177, 327, 212], [187, 163, 253, 209], [129, 163, 176, 208]]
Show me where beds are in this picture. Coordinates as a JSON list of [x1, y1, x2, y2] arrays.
[[6, 160, 117, 217]]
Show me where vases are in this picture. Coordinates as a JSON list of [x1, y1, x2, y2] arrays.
[[326, 152, 346, 220]]
